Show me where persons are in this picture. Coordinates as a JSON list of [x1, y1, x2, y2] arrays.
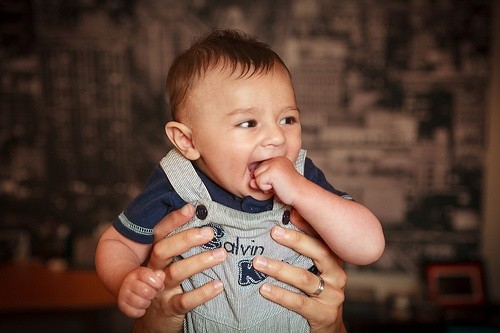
[[133, 202, 347, 333], [94, 29, 387, 333]]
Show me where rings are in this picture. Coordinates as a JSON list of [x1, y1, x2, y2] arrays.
[[307, 274, 325, 297]]
